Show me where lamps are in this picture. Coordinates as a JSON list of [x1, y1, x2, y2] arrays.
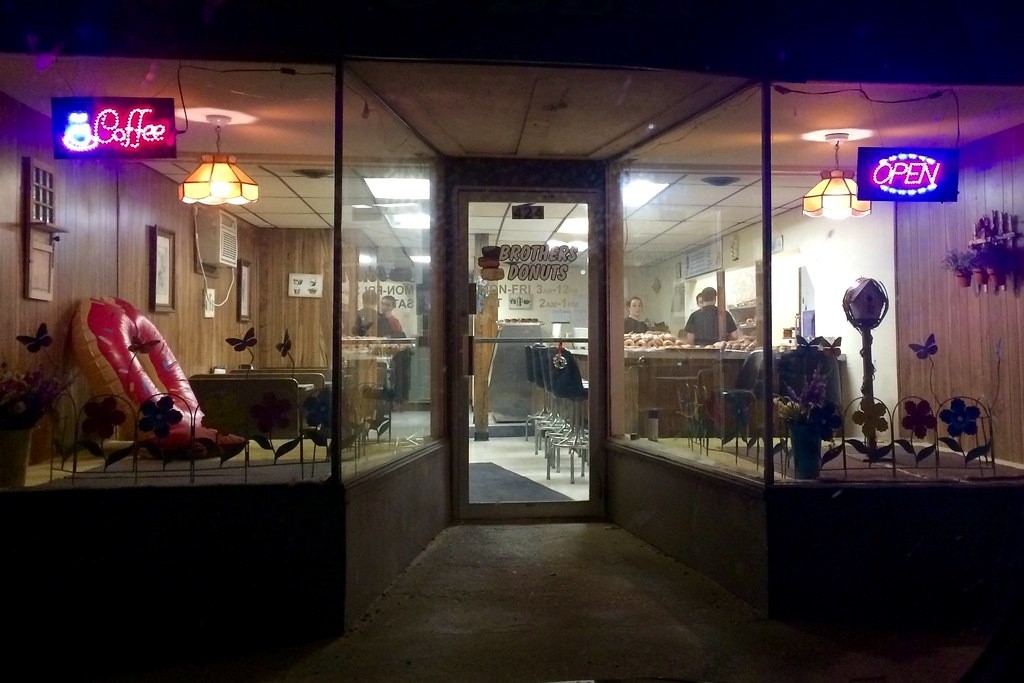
[[177, 114, 259, 207], [802, 133, 871, 219]]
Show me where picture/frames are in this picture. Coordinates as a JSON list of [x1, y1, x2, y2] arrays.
[[238, 257, 253, 322], [151, 223, 177, 314]]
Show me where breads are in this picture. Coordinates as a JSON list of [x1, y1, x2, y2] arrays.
[[705, 340, 757, 351]]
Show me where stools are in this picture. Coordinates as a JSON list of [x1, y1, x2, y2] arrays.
[[522, 345, 588, 484]]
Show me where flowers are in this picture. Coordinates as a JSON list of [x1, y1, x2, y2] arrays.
[[776, 351, 829, 424]]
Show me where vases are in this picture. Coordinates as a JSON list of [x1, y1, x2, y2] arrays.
[[791, 423, 822, 479]]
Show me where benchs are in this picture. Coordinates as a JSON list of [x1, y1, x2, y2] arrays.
[[188, 359, 385, 480]]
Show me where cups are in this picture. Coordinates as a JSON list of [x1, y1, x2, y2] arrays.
[[573, 327, 588, 350]]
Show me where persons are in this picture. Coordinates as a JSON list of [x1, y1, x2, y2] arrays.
[[379, 295, 406, 338], [684, 287, 739, 345], [624, 297, 648, 335], [696, 293, 702, 308], [358, 290, 391, 430]]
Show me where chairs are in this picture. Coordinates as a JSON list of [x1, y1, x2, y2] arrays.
[[634, 347, 847, 476]]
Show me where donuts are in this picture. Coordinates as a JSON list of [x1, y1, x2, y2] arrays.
[[623, 333, 685, 347]]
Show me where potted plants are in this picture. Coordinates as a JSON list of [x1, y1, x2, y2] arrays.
[[940, 240, 1011, 287]]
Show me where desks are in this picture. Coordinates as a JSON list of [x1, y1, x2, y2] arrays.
[[324, 380, 333, 388], [342, 337, 415, 345], [298, 383, 315, 393]]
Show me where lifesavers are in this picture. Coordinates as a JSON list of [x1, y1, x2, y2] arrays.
[[71, 295, 204, 435]]
